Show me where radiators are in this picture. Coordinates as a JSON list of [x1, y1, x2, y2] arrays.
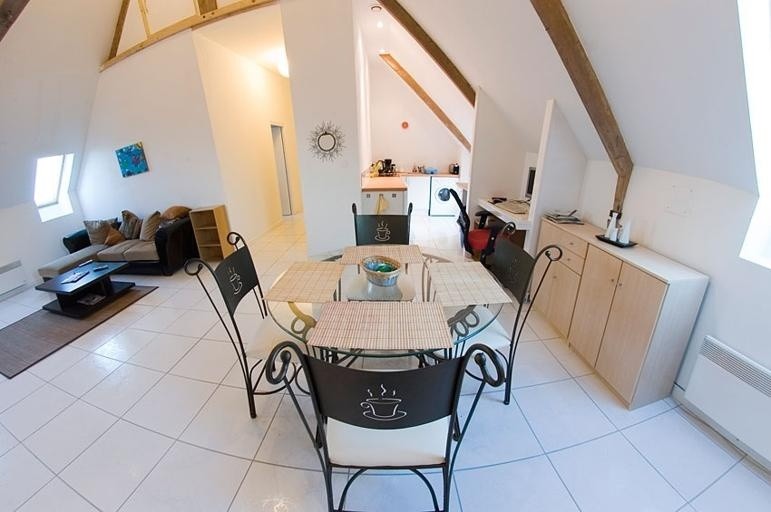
[[0, 260, 26, 295], [685, 335, 771, 471]]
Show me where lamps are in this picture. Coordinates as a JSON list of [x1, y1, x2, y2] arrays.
[[270, 45, 289, 78]]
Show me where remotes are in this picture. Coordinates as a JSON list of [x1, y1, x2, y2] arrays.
[[78, 260, 93, 267], [93, 265, 109, 271]]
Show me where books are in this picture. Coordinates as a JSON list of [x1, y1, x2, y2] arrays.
[[61, 270, 89, 283]]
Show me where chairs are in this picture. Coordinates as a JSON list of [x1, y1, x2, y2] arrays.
[[418, 222, 563, 405], [352, 203, 413, 245], [184, 232, 322, 419], [266, 341, 505, 511], [449, 188, 509, 267]]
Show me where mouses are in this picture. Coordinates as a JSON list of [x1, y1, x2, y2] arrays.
[[493, 199, 503, 203]]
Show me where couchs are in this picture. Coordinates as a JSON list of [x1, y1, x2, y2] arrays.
[[38, 217, 200, 282]]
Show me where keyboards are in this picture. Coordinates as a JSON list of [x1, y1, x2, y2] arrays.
[[496, 202, 527, 214]]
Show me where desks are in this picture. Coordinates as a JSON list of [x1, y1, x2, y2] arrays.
[[267, 244, 504, 448], [478, 198, 529, 232]]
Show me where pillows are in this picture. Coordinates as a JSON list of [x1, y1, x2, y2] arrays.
[[104, 223, 125, 245], [139, 211, 161, 240], [161, 206, 192, 219], [84, 217, 118, 244], [119, 210, 142, 240]]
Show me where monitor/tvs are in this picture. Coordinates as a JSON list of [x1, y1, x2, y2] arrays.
[[525, 167, 536, 201]]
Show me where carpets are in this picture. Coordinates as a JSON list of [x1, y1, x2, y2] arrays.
[[0, 286, 158, 379]]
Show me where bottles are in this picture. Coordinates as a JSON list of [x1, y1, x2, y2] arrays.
[[604, 211, 631, 244]]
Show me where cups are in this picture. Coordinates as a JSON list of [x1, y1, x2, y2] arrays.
[[384, 159, 392, 167]]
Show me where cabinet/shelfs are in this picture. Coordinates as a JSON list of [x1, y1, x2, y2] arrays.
[[568, 244, 709, 410], [530, 220, 588, 340], [189, 205, 234, 259]]
